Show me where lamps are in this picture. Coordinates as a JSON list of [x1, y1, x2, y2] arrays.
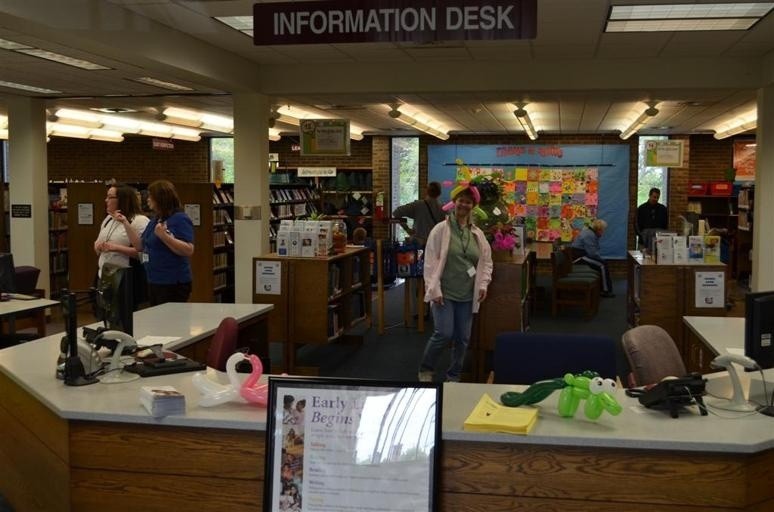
[[619, 100, 665, 142], [269, 103, 366, 142], [155, 102, 282, 143], [511, 102, 541, 142], [50, 108, 200, 142], [387, 98, 453, 141]]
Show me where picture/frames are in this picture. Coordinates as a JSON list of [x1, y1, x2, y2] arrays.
[[733, 139, 761, 186]]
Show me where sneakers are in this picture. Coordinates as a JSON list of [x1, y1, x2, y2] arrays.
[[418, 371, 432, 383]]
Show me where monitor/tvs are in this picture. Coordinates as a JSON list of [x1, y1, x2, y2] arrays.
[[0, 252, 17, 302], [744, 290, 774, 417], [97, 262, 134, 337]]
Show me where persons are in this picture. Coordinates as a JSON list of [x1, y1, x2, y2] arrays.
[[109, 179, 197, 309], [391, 178, 449, 250], [279, 395, 303, 511], [416, 185, 493, 384], [90, 181, 152, 283], [633, 185, 671, 244], [564, 219, 620, 299]]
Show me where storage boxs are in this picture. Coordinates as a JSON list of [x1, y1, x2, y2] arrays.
[[687, 181, 707, 196], [711, 181, 732, 195]]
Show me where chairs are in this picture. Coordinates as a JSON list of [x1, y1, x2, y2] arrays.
[[623, 325, 693, 389], [550, 241, 603, 322], [492, 331, 617, 393], [208, 316, 240, 372]]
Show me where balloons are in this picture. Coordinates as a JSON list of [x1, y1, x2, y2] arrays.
[[200, 348, 272, 410], [496, 367, 624, 423]]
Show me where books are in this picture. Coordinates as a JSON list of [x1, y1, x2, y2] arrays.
[[48, 184, 70, 312], [213, 183, 320, 305]]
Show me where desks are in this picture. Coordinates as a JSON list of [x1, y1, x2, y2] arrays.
[[0, 292, 60, 341]]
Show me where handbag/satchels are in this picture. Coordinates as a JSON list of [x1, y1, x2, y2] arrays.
[[129, 260, 149, 305]]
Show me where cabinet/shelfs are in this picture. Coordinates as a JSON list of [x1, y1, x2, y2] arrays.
[[689, 194, 752, 283], [478, 252, 538, 334], [47, 181, 234, 301], [251, 244, 372, 345], [626, 252, 731, 374], [270, 167, 373, 249]]
